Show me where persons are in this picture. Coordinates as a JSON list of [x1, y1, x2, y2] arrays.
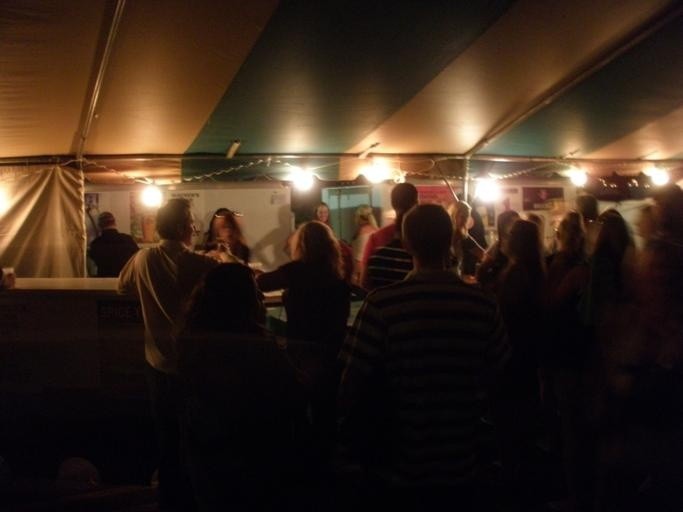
[[115, 181, 683, 510], [87, 211, 139, 277]]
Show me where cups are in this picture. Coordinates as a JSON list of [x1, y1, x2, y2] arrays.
[[2, 267, 14, 287]]
